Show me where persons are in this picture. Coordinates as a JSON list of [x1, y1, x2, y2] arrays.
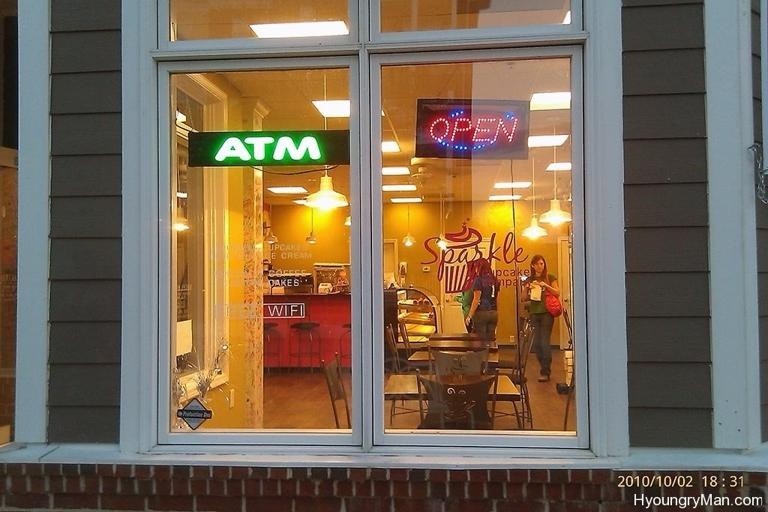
[[453, 261, 478, 333], [521, 255, 561, 382], [464, 259, 500, 335]]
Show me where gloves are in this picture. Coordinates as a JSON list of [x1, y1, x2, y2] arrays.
[[464, 316, 474, 334]]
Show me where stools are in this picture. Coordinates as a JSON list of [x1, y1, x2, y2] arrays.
[[264, 323, 352, 377]]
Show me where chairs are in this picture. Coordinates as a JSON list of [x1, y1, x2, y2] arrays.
[[320, 310, 536, 429]]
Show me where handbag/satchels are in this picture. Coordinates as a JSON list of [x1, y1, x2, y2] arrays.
[[545, 290, 562, 317]]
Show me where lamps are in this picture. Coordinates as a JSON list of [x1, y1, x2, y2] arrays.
[[290, 69, 349, 213], [516, 126, 572, 242]]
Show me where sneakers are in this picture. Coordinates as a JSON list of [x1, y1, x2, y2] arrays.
[[537, 374, 549, 382]]
